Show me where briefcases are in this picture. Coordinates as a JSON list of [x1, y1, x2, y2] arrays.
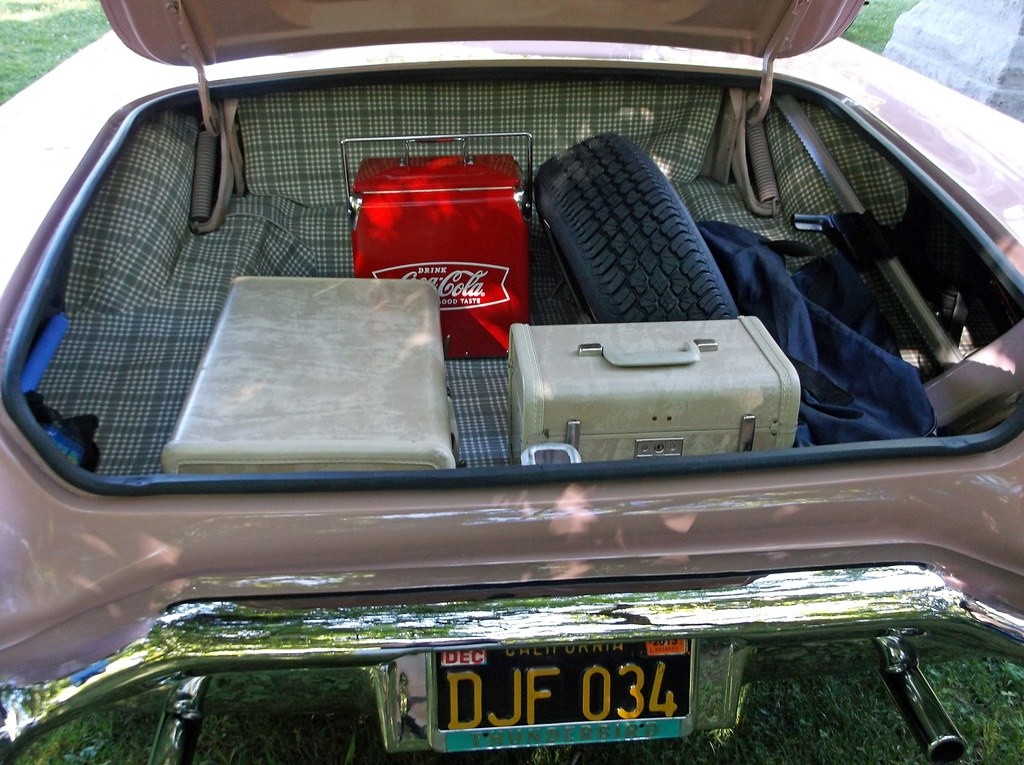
[[341, 133, 535, 360]]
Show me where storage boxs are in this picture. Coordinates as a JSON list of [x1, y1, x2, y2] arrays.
[[507, 314, 800, 465], [160, 274, 454, 474]]
